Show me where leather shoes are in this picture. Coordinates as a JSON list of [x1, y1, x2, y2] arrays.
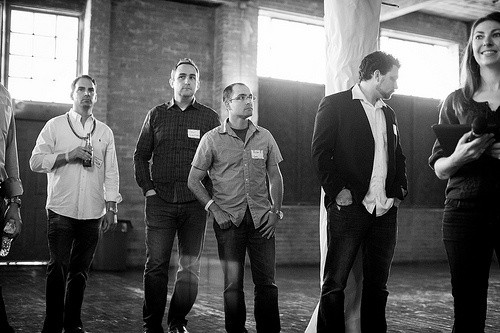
[[169, 324, 189, 333], [145, 326, 164, 333]]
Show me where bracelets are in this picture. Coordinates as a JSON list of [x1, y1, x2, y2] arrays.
[[7, 196, 22, 208], [106, 206, 119, 214], [205, 200, 214, 211]]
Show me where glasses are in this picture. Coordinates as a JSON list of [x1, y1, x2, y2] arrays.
[[230, 95, 258, 102]]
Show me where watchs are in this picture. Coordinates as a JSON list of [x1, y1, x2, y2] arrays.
[[270, 208, 283, 220]]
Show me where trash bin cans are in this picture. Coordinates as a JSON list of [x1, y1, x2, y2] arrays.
[[94, 221, 131, 269]]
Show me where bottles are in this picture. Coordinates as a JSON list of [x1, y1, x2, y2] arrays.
[[0, 218, 15, 257], [82, 132, 94, 167]]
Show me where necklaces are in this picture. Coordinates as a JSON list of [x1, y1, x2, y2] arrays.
[[66, 112, 96, 139]]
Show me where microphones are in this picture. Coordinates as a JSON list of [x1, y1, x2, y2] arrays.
[[470, 116, 488, 141]]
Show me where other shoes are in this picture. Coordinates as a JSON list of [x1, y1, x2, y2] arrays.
[[64, 326, 94, 333]]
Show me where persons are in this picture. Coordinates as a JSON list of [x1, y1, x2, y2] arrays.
[[427, 13, 499, 333], [133, 57, 221, 333], [311, 51, 406, 333], [29, 74, 124, 333], [0, 85, 24, 257], [188, 82, 288, 333]]
